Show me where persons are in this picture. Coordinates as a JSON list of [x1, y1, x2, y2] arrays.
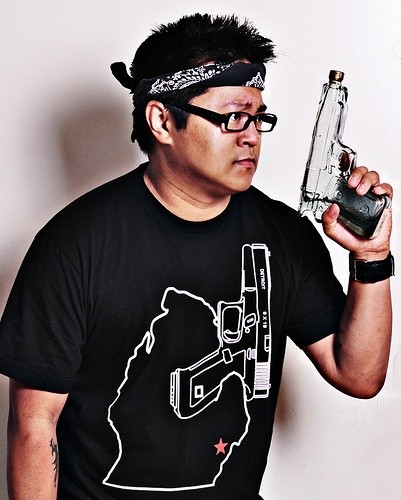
[[0, 11, 394, 500]]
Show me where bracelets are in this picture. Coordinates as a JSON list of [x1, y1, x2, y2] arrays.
[[349, 249, 396, 285]]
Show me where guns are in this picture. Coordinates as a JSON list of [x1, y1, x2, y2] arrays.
[[297, 70, 393, 241]]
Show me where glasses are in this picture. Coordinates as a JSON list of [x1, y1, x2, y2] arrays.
[[161, 99, 278, 132]]
